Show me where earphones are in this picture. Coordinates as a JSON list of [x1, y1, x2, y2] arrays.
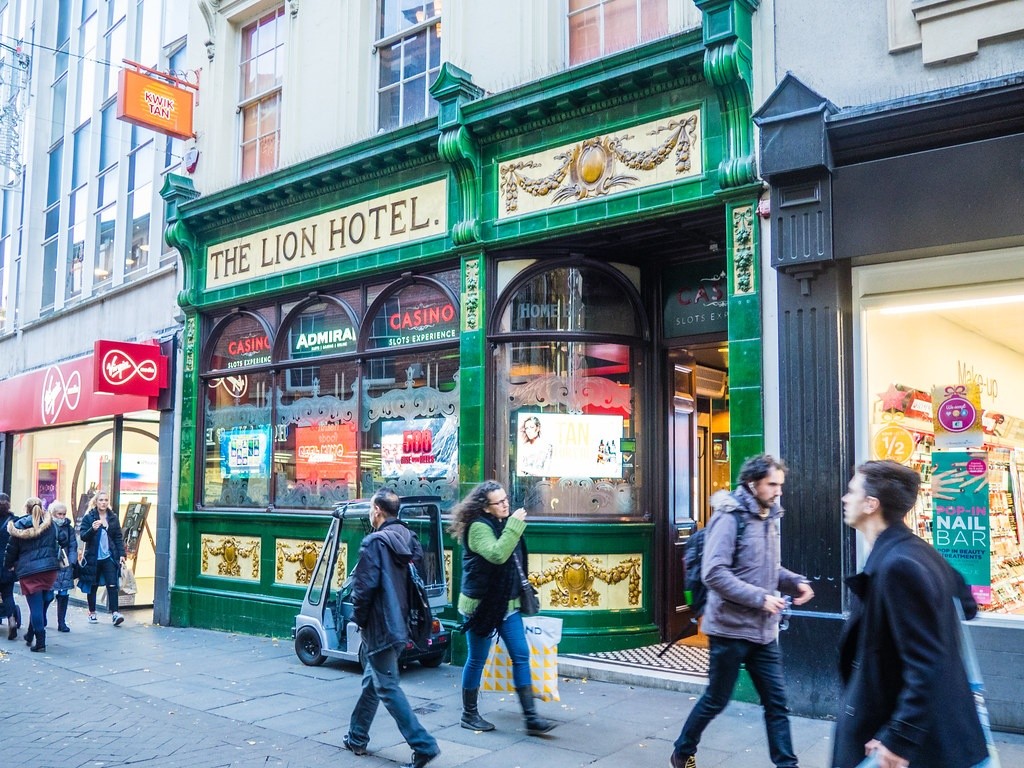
[[748, 482, 754, 489]]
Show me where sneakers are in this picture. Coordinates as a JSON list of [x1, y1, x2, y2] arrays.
[[668, 748, 697, 768], [112, 613, 125, 626], [88, 613, 98, 624]]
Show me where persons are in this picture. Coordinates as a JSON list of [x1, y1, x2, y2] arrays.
[[829, 457, 993, 768], [669, 454, 817, 768], [3, 496, 60, 653], [445, 480, 563, 733], [77, 491, 128, 625], [42, 499, 78, 633], [342, 488, 442, 768], [0, 491, 20, 640]]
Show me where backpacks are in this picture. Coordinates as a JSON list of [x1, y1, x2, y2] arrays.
[[683, 509, 744, 591]]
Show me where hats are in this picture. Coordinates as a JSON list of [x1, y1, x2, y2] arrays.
[[0, 492, 10, 502]]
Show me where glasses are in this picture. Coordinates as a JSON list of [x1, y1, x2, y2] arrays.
[[488, 494, 511, 505]]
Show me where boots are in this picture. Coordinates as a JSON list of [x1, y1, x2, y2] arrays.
[[43, 598, 54, 627], [55, 594, 70, 632], [514, 685, 558, 735], [8, 616, 18, 640], [23, 621, 34, 647], [30, 630, 46, 652], [460, 687, 496, 732]]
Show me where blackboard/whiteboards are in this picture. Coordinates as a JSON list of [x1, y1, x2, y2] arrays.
[[122, 502, 151, 559]]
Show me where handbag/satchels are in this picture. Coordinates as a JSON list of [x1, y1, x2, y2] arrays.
[[479, 615, 566, 702], [57, 547, 70, 569], [521, 578, 540, 616], [407, 560, 434, 651], [117, 562, 137, 596]]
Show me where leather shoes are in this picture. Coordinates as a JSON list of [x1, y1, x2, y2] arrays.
[[399, 751, 441, 768], [342, 735, 367, 755]]
